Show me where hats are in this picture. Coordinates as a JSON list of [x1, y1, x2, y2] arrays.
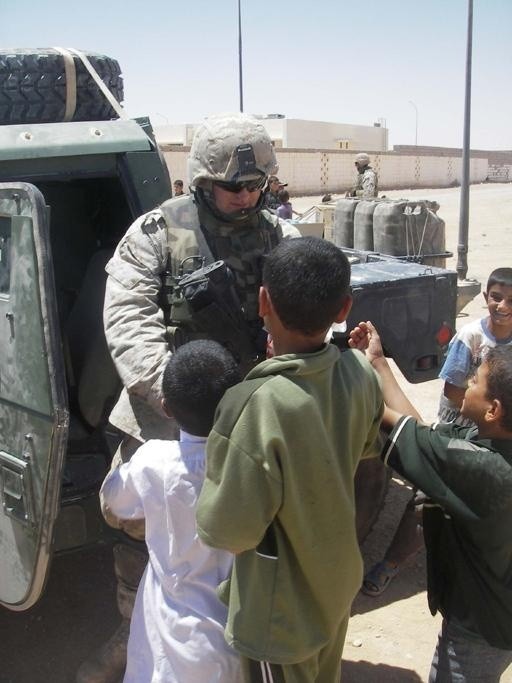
[[278, 180, 288, 187]]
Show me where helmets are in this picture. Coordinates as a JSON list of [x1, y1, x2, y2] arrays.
[[188, 113, 279, 185], [354, 153, 370, 167]]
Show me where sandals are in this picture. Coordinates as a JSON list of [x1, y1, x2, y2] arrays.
[[361, 557, 400, 597]]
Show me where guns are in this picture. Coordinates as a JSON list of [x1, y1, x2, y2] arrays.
[[179, 260, 243, 330]]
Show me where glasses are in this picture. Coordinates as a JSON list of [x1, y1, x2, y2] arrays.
[[213, 176, 268, 193]]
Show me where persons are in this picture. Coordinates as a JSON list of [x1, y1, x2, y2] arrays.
[[191, 235, 389, 680], [264, 174, 282, 208], [96, 335, 263, 683], [345, 153, 379, 198], [275, 190, 303, 219], [72, 112, 290, 683], [434, 264, 511, 435], [172, 178, 185, 197], [345, 317, 512, 682], [276, 181, 288, 191]]
